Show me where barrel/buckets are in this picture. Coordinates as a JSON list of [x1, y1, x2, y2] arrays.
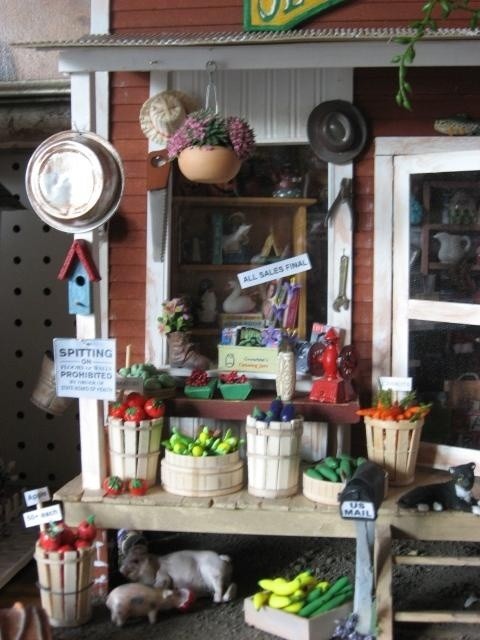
[[28, 352, 74, 417]]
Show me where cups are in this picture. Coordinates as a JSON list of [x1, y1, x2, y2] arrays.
[[422, 273, 437, 298]]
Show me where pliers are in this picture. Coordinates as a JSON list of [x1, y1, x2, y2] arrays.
[[323, 178, 358, 232]]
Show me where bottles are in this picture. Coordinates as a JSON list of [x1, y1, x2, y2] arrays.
[[450, 191, 478, 225], [409, 193, 424, 227]]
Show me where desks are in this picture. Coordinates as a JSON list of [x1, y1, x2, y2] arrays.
[[49, 451, 480, 640]]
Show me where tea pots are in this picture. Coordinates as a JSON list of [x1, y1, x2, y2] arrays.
[[434, 231, 472, 263]]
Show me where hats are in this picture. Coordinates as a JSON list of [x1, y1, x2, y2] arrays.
[[137, 90, 202, 145]]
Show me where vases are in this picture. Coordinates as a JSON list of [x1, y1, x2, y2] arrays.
[[161, 327, 197, 371], [177, 136, 245, 187]]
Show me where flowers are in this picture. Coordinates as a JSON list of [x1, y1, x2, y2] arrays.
[[161, 102, 260, 163], [151, 292, 195, 337]]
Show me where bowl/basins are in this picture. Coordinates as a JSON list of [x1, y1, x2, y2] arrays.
[[23, 129, 126, 235]]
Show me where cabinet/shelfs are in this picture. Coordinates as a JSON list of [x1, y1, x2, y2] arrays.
[[161, 185, 319, 358], [406, 170, 479, 303]]
